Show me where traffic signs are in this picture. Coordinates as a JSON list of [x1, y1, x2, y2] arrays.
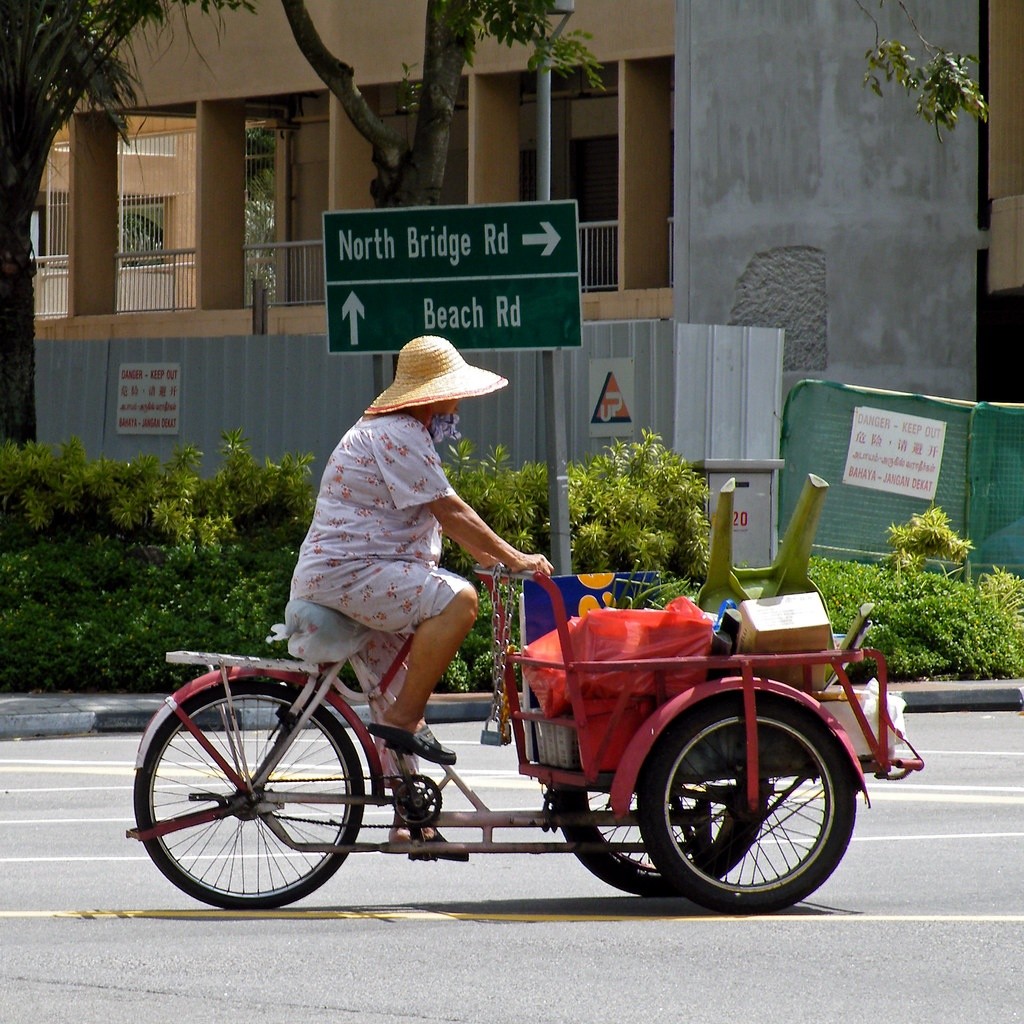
[[323, 273, 585, 356], [321, 198, 582, 283]]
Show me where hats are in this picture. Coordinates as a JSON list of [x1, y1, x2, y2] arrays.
[[363, 335, 509, 415]]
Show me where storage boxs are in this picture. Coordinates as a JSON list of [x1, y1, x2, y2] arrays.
[[535, 720, 580, 768], [586, 699, 652, 770], [737, 591, 831, 691], [810, 691, 904, 755]]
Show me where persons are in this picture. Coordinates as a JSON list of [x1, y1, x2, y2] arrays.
[[289, 335, 554, 863]]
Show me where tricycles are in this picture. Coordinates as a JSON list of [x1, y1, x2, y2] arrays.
[[119, 559, 925, 916]]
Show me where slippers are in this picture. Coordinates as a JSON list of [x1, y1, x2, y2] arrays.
[[368, 722, 457, 765], [381, 832, 469, 862]]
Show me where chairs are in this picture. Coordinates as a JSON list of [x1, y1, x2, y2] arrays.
[[696, 473, 875, 692]]
